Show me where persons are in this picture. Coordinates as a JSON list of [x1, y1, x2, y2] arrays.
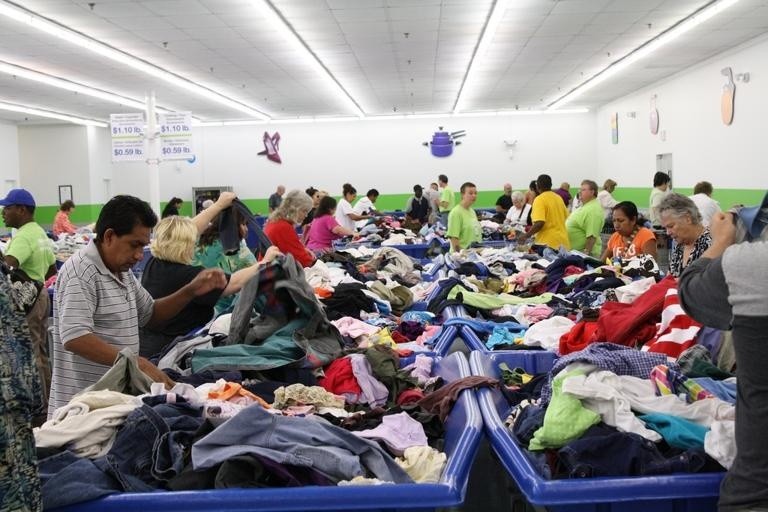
[[138, 192, 285, 360], [495, 170, 729, 294], [0, 249, 46, 511], [436, 174, 454, 225], [1, 188, 57, 415], [257, 181, 379, 267], [48, 194, 226, 418], [448, 182, 483, 253], [692, 203, 768, 510], [54, 199, 77, 239], [197, 201, 257, 315], [405, 185, 432, 221], [161, 197, 184, 220], [424, 183, 440, 216]]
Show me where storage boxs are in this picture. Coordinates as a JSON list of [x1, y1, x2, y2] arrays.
[[132, 206, 536, 350], [469, 349, 733, 512], [72, 348, 483, 512]]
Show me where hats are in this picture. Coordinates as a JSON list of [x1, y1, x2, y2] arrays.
[[413, 185, 425, 190], [605, 179, 616, 188], [0, 189, 34, 205]]
